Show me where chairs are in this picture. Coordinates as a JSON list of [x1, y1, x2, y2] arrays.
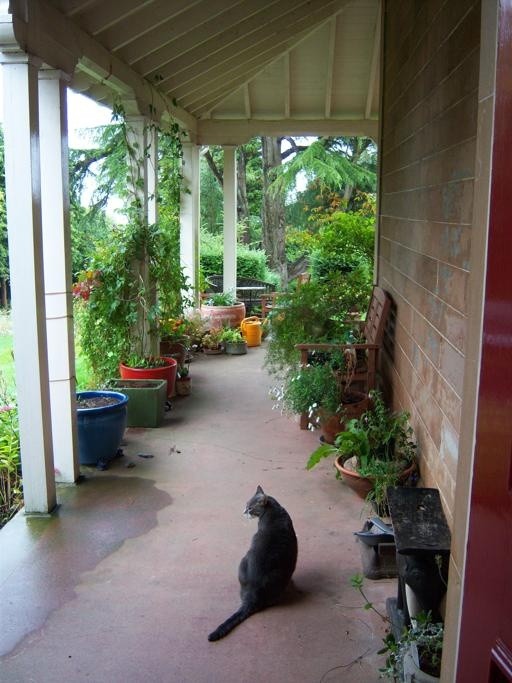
[[205, 275, 276, 317], [261, 274, 312, 318], [294, 284, 392, 430]]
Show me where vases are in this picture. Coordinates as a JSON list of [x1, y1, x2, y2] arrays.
[[319, 391, 372, 446]]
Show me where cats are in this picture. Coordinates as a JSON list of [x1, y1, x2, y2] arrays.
[[208, 484, 298, 643]]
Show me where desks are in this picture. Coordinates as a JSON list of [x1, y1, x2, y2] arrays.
[[236, 287, 267, 318]]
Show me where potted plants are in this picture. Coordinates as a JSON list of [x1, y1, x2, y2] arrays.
[[304, 388, 420, 499], [349, 571, 445, 683], [74, 220, 248, 473]]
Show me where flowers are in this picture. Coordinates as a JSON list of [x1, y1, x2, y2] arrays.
[[268, 350, 348, 431]]
